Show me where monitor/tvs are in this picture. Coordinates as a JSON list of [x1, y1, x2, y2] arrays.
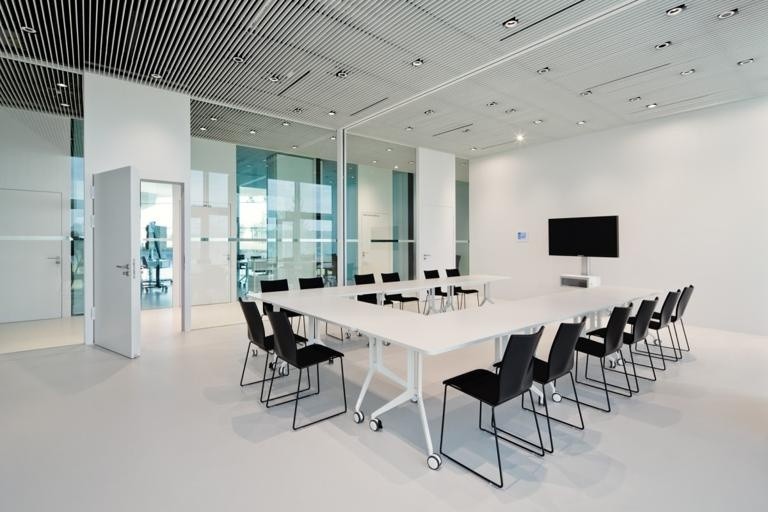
[[548, 216, 619, 258]]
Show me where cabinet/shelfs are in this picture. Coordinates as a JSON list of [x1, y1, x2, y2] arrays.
[[560, 274, 598, 287]]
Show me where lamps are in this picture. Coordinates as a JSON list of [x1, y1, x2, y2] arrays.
[[331, 136, 336, 140], [329, 109, 336, 115], [646, 103, 656, 108], [534, 120, 543, 125], [538, 68, 548, 73], [338, 73, 348, 77], [656, 43, 669, 49], [413, 61, 422, 66], [739, 60, 753, 65], [152, 73, 161, 79], [718, 11, 735, 19], [233, 56, 243, 63], [283, 121, 289, 125], [668, 8, 682, 15], [576, 120, 585, 125], [295, 109, 301, 113], [581, 91, 592, 96], [269, 77, 279, 82], [489, 103, 496, 107], [505, 21, 517, 28], [681, 70, 693, 75], [21, 26, 36, 33]]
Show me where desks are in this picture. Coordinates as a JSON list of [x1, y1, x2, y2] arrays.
[[144, 260, 169, 292], [245, 275, 660, 470]]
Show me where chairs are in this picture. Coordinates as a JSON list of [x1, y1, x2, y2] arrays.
[[446, 268, 480, 309], [381, 272, 420, 313], [585, 296, 659, 393], [259, 278, 308, 347], [238, 296, 311, 403], [265, 308, 348, 431], [438, 324, 544, 488], [354, 273, 393, 311], [636, 284, 694, 359], [423, 270, 458, 315], [554, 302, 634, 414], [626, 288, 679, 371], [298, 277, 344, 342], [491, 315, 584, 454]]
